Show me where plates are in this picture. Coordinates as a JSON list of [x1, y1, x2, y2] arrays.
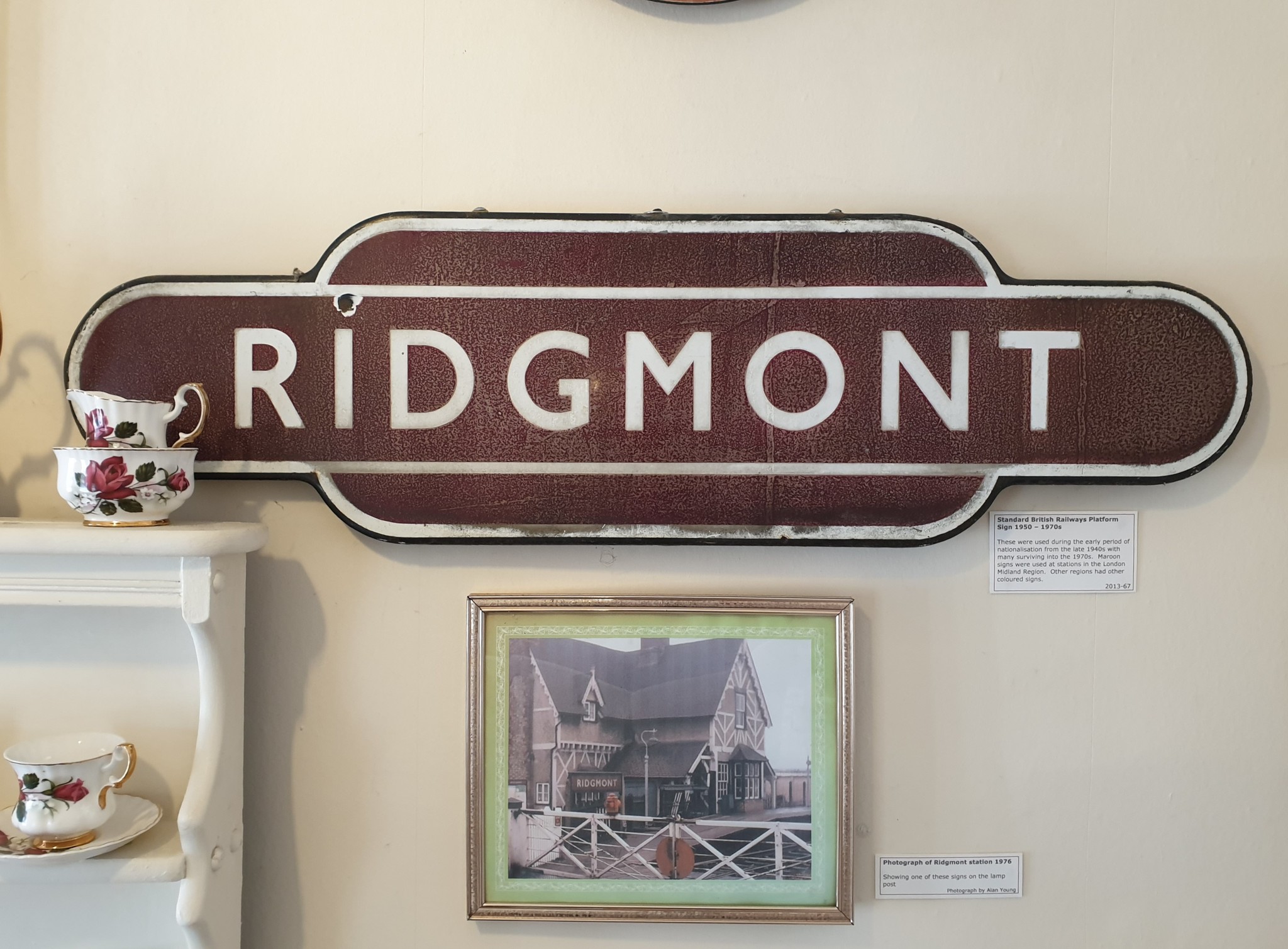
[[1, 792, 162, 860]]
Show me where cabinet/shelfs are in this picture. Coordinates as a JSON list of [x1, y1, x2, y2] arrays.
[[1, 516, 261, 949]]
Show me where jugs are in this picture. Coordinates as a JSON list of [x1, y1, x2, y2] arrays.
[[66, 382, 209, 448]]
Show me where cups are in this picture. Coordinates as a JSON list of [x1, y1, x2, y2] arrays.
[[3, 731, 137, 850]]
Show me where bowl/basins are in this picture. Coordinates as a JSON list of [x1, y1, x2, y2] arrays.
[[51, 446, 199, 528]]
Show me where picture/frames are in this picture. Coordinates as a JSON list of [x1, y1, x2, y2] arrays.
[[467, 592, 856, 925]]
